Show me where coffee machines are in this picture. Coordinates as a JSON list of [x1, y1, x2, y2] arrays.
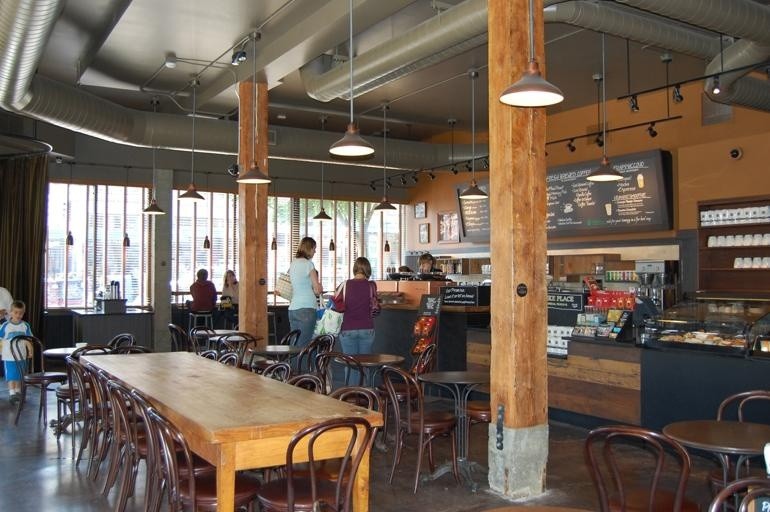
[[635, 260, 681, 312]]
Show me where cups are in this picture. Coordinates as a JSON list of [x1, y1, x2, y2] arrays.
[[605, 204, 612, 216], [733, 257, 770, 268], [637, 174, 645, 189], [708, 234, 770, 247]]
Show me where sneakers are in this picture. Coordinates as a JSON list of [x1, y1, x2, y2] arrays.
[[8, 393, 22, 404]]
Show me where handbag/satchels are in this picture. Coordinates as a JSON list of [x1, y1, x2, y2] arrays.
[[314, 310, 344, 338], [274, 272, 294, 300], [369, 296, 381, 320]]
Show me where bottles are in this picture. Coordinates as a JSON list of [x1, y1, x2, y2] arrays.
[[574, 305, 608, 337]]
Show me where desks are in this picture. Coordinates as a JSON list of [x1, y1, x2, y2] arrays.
[[69, 308, 155, 352]]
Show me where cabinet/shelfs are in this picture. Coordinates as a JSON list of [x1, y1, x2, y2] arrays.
[[697, 194, 770, 293], [640, 300, 770, 468], [437, 254, 679, 328]]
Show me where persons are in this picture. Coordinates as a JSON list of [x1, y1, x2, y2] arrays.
[[335, 256, 378, 387], [0, 285, 14, 325], [287, 237, 323, 372], [0, 300, 34, 402], [417, 253, 442, 272], [218, 269, 239, 326], [186, 269, 217, 314]]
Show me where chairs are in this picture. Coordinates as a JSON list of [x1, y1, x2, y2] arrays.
[[582, 389, 770, 511]]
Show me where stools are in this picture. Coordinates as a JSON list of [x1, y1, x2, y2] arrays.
[[187, 311, 279, 347]]
